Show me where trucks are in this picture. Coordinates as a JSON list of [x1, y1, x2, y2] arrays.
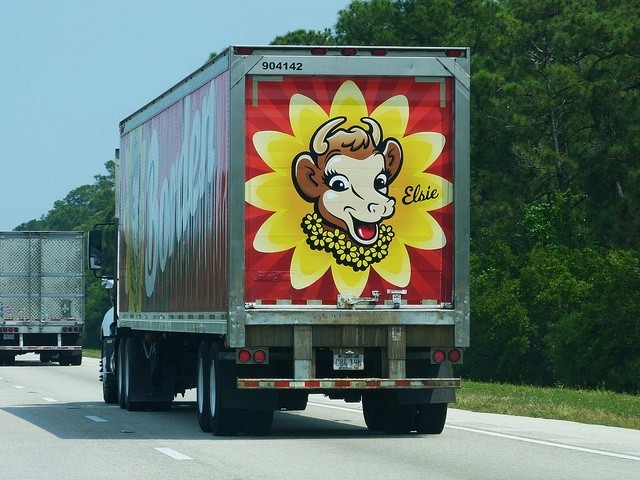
[[87, 44, 472, 437], [1, 229, 87, 366]]
